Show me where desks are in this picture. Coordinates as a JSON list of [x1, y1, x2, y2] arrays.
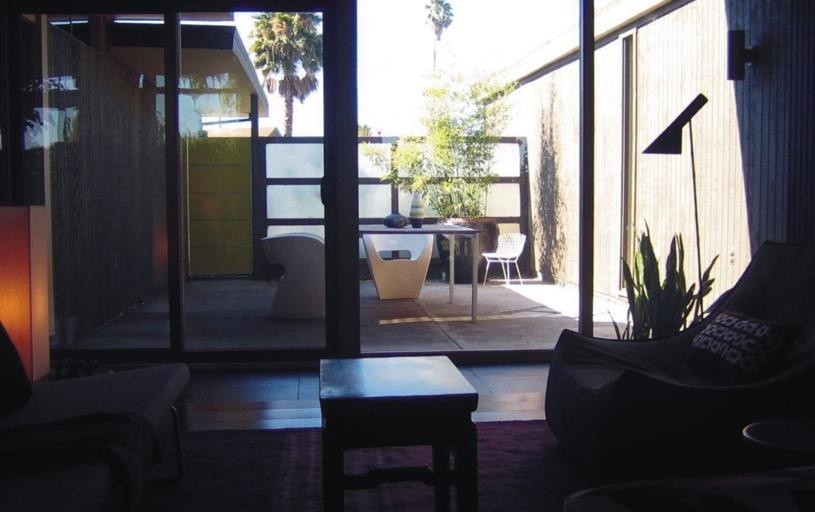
[[358, 224, 482, 323]]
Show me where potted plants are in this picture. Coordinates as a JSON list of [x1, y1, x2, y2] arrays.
[[357, 66, 526, 284]]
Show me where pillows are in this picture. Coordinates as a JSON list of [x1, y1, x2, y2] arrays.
[[687, 305, 804, 385]]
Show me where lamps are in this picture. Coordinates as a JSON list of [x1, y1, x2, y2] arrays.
[[0, 205, 50, 383]]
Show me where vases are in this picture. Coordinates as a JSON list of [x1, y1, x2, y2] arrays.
[[409, 191, 426, 228]]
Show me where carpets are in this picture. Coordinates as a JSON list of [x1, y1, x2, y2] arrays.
[[107, 419, 595, 512]]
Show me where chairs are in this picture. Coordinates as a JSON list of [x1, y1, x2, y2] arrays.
[[481, 233, 527, 286], [260, 233, 434, 320], [0, 321, 191, 512]]
